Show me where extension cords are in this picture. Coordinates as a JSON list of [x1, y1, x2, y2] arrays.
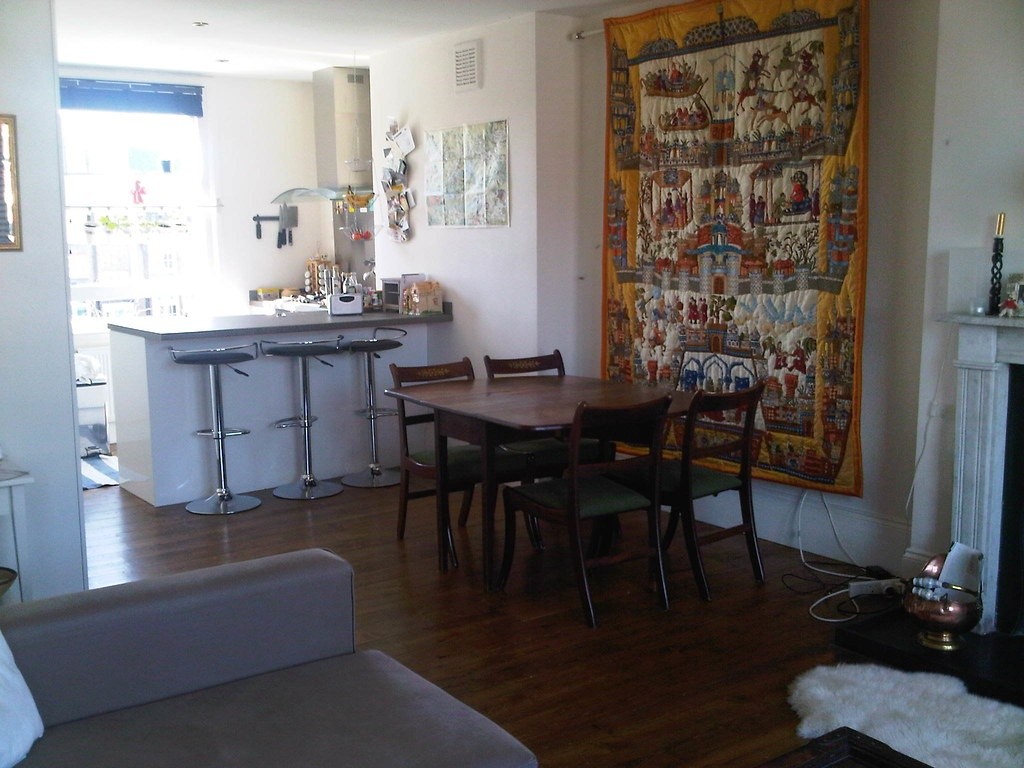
[[848, 578, 905, 598]]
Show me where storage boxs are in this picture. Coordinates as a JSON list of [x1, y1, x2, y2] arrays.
[[380, 278, 400, 313]]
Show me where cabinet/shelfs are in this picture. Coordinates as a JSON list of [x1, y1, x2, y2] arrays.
[[0, 467, 35, 609], [934, 313, 1024, 639]]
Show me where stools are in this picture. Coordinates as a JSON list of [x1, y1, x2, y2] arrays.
[[170, 341, 262, 514], [260, 334, 345, 501], [340, 327, 408, 488]]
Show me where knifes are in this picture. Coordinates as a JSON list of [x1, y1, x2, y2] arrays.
[[276, 202, 299, 249]]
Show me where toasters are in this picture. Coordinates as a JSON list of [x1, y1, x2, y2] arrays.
[[327, 293, 363, 317]]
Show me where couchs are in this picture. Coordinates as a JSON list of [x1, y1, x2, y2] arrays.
[[0, 546, 542, 768]]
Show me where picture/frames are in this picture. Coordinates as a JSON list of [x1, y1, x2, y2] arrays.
[[0, 114, 22, 250], [1006, 272, 1024, 318]]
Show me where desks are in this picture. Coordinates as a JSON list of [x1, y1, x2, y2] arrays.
[[106, 309, 456, 507], [384, 375, 702, 593]]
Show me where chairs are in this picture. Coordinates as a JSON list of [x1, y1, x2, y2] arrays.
[[483, 349, 626, 553], [388, 357, 546, 569], [601, 380, 770, 601], [496, 392, 673, 630]]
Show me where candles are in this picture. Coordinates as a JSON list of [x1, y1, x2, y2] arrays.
[[995, 212, 1006, 236]]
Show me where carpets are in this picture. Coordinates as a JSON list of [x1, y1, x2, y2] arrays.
[[787, 663, 1024, 768], [80, 455, 120, 490]]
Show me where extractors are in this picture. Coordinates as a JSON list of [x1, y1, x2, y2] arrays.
[[270, 181, 373, 203]]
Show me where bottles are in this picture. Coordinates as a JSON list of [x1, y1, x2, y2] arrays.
[[332, 265, 382, 309]]
